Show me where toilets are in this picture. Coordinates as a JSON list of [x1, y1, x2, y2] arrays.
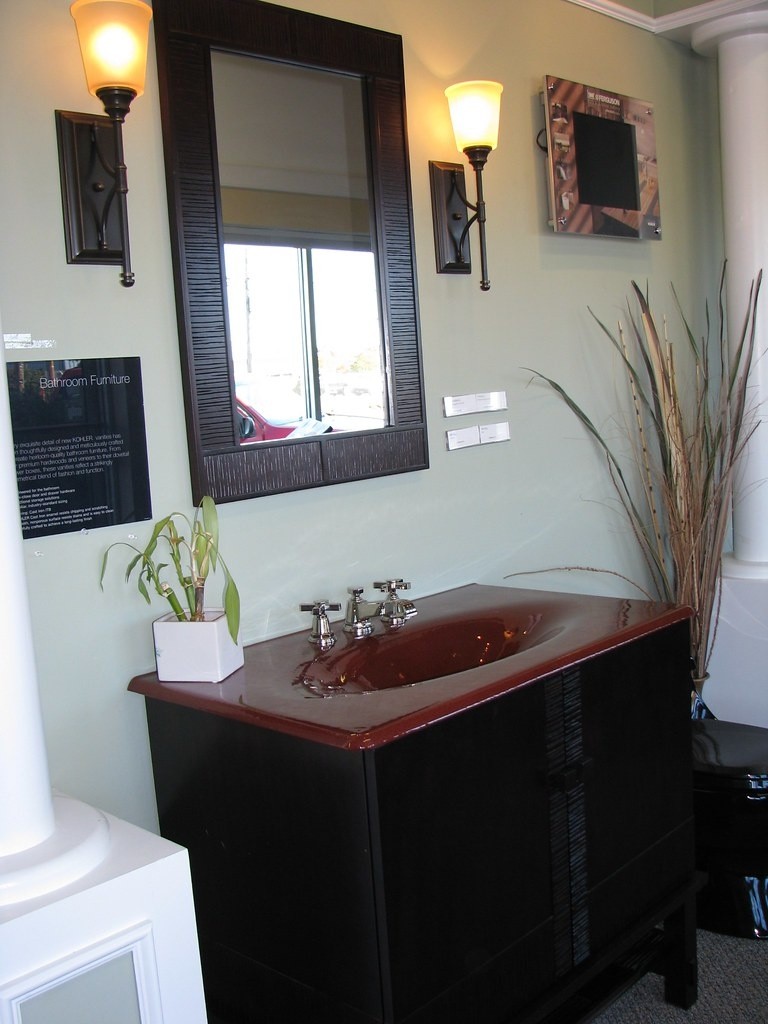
[[690, 718, 768, 941]]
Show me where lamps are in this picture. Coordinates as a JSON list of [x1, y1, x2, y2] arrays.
[[54, 0, 154, 290], [426, 80, 504, 291]]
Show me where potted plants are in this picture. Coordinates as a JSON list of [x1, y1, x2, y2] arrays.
[[99, 495, 247, 683]]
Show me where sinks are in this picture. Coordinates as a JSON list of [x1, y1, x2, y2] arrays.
[[303, 602, 581, 696]]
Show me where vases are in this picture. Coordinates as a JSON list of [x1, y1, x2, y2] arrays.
[[691, 672, 717, 720]]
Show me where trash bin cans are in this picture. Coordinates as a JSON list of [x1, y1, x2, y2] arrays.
[[692, 718, 768, 941]]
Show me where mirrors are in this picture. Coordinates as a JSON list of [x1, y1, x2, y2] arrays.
[[152, 0, 431, 508]]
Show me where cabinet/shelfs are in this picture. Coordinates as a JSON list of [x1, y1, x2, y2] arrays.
[[144, 620, 710, 1024]]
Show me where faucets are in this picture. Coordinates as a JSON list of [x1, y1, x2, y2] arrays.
[[342, 586, 417, 639]]
